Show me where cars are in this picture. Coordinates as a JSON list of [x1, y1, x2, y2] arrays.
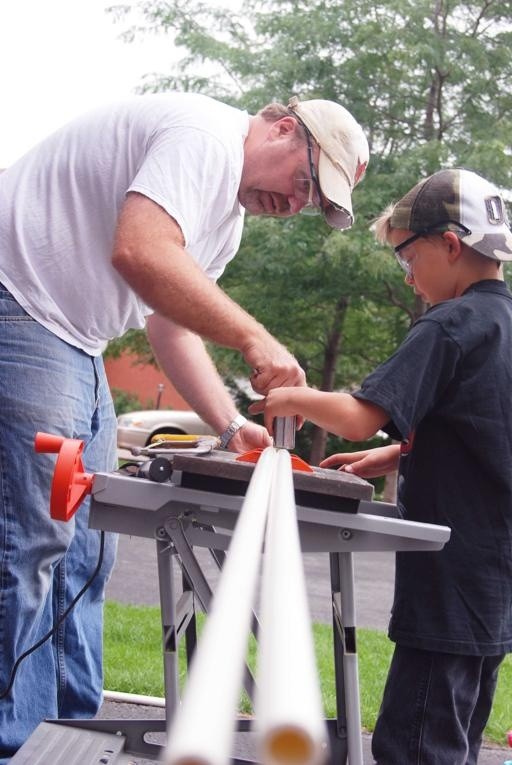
[[116, 410, 220, 457]]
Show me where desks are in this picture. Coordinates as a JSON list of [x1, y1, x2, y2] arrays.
[[87, 471, 450, 765]]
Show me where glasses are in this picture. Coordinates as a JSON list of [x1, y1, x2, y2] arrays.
[[393, 220, 471, 277], [293, 130, 323, 216]]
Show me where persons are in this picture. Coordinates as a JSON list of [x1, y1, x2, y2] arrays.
[[247, 170, 512, 764], [1, 94, 369, 764]]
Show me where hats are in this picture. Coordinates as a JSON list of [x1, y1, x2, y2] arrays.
[[287, 96, 370, 230], [390, 169, 512, 261]]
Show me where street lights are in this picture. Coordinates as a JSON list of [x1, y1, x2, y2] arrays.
[[156, 383, 165, 411]]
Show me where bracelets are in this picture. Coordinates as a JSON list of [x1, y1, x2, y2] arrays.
[[218, 414, 246, 449]]
